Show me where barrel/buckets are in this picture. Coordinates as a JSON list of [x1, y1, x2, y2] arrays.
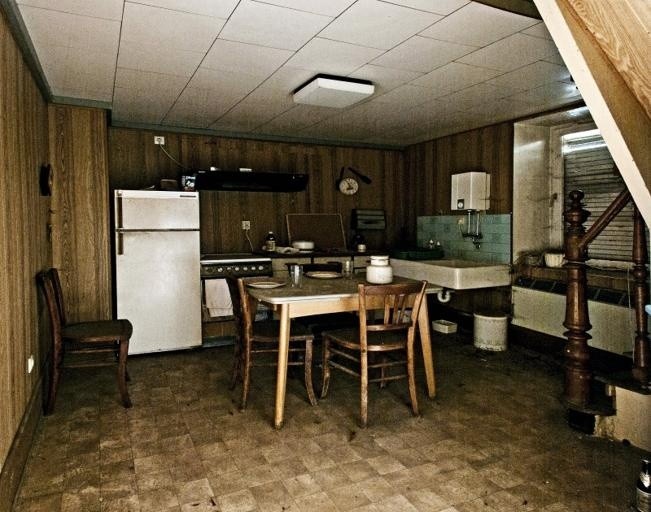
[[472, 310, 509, 352]]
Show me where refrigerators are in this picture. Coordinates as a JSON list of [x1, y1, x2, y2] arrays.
[[113, 188, 203, 356]]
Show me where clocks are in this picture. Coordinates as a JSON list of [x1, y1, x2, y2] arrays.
[[338, 177, 358, 196]]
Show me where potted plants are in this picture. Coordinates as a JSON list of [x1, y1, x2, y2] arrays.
[[515, 247, 566, 269]]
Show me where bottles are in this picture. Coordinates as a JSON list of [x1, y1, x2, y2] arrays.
[[266, 231, 275, 256], [634, 458, 651, 512]]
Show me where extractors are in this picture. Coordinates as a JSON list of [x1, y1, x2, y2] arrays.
[[195, 167, 308, 193]]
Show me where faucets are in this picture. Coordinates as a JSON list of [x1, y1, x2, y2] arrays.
[[464, 230, 481, 248]]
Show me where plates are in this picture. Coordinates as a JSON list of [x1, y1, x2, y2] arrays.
[[306, 270, 344, 279], [245, 281, 286, 290]]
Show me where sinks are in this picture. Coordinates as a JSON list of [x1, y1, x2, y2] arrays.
[[424, 259, 511, 291]]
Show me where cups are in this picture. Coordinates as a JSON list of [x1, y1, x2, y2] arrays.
[[341, 260, 353, 279], [290, 264, 303, 287]]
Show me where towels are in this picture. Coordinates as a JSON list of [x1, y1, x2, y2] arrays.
[[203, 278, 234, 317]]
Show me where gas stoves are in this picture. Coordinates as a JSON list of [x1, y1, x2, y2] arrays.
[[200, 253, 273, 279]]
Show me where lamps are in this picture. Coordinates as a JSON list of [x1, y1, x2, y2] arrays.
[[290, 72, 375, 108]]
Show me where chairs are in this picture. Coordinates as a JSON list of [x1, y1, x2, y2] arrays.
[[229, 261, 428, 431], [34, 267, 133, 417]]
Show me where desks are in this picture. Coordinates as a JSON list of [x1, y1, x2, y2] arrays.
[[245, 271, 443, 401]]
